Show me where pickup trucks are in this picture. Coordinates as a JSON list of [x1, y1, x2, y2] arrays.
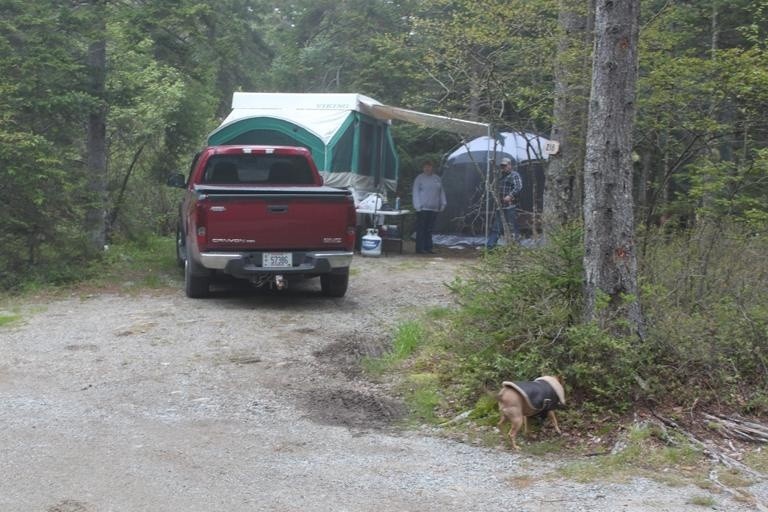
[[176, 145, 357, 297]]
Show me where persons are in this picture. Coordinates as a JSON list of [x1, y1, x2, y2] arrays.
[[411, 160, 447, 255], [486, 157, 523, 250]]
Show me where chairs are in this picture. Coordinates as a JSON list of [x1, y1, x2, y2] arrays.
[[268, 161, 299, 184], [208, 161, 240, 183]]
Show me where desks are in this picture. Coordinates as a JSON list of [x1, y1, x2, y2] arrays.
[[355, 209, 411, 258]]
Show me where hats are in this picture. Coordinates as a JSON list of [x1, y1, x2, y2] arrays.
[[499, 157, 512, 166]]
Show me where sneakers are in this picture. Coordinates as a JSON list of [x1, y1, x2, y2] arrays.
[[415, 248, 434, 256]]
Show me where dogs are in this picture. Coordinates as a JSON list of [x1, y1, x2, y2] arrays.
[[481, 372, 567, 452]]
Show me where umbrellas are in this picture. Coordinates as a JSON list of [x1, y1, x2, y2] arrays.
[[443, 129, 550, 168]]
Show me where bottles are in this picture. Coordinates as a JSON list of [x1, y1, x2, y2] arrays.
[[395, 197, 400, 210]]
